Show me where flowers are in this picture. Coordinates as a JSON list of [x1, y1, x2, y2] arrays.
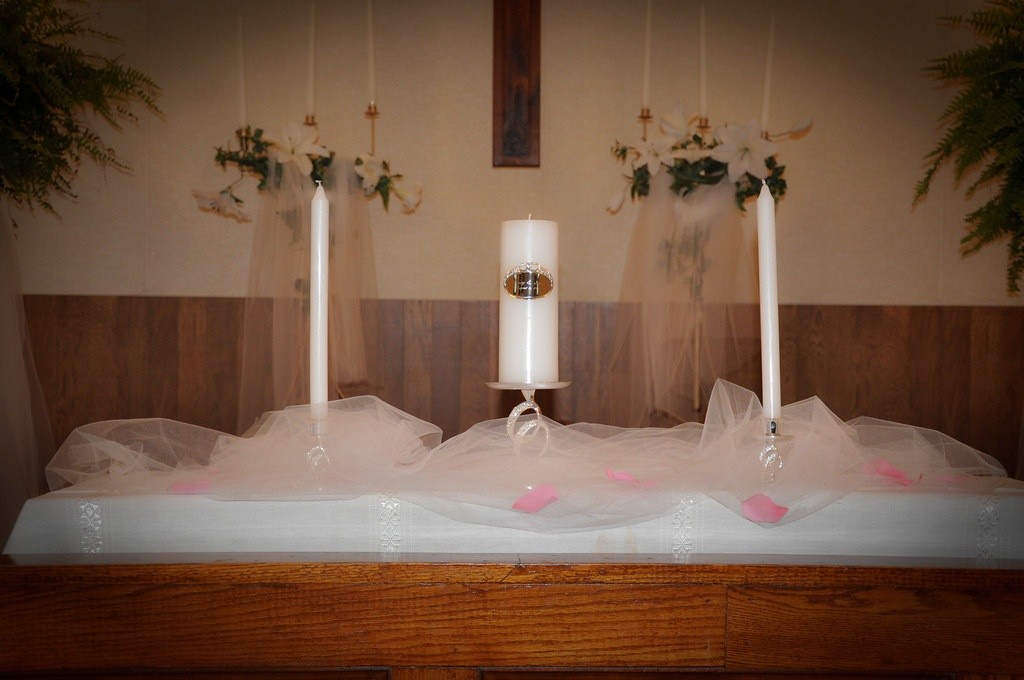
[[603, 114, 815, 212], [193, 121, 424, 222]]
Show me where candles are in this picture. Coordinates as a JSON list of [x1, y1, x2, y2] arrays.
[[309, 180, 330, 422], [307, 0, 316, 115], [237, 14, 248, 128], [366, 0, 376, 106], [499, 213, 560, 383], [641, 0, 652, 106], [756, 178, 781, 419], [761, 13, 777, 130], [698, 4, 707, 118]]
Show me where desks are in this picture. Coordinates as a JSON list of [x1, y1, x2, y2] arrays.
[[0, 552, 1024, 680]]
